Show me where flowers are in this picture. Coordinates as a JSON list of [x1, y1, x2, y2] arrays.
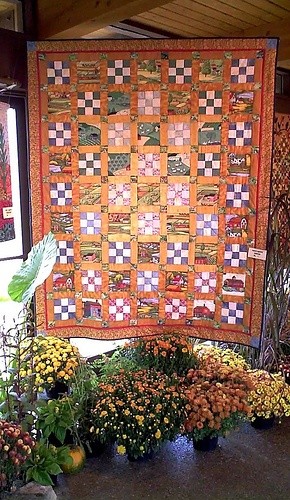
[[0, 333, 290, 500]]
